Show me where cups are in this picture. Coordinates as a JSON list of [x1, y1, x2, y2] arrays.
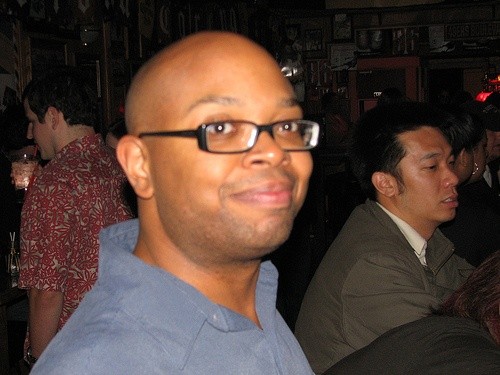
[[12, 155, 38, 190]]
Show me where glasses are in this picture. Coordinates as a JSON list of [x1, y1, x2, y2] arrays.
[[137, 119, 319, 154]]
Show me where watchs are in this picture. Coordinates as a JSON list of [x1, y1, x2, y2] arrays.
[[25, 346, 38, 364]]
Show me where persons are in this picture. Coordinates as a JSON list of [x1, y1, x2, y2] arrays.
[[0, 67, 500, 375], [30, 30, 321, 375]]
